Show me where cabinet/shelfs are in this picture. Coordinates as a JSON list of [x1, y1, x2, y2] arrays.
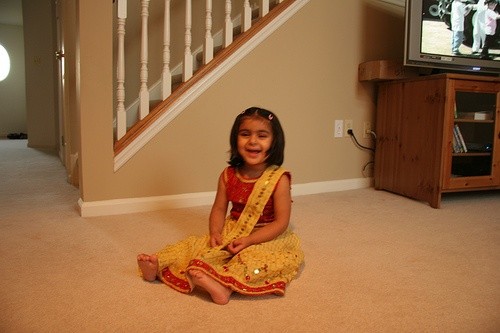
[[374, 72, 500, 209]]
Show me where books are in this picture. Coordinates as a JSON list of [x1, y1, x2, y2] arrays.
[[452, 124, 467, 153]]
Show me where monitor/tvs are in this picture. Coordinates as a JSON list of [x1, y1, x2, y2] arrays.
[[402, 0, 500, 74]]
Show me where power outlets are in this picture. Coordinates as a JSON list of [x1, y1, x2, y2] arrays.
[[363, 121, 371, 139], [335, 120, 343, 138], [345, 120, 352, 137]]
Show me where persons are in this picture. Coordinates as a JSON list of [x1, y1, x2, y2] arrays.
[[451, 0, 500, 57], [138, 107, 305, 305]]
